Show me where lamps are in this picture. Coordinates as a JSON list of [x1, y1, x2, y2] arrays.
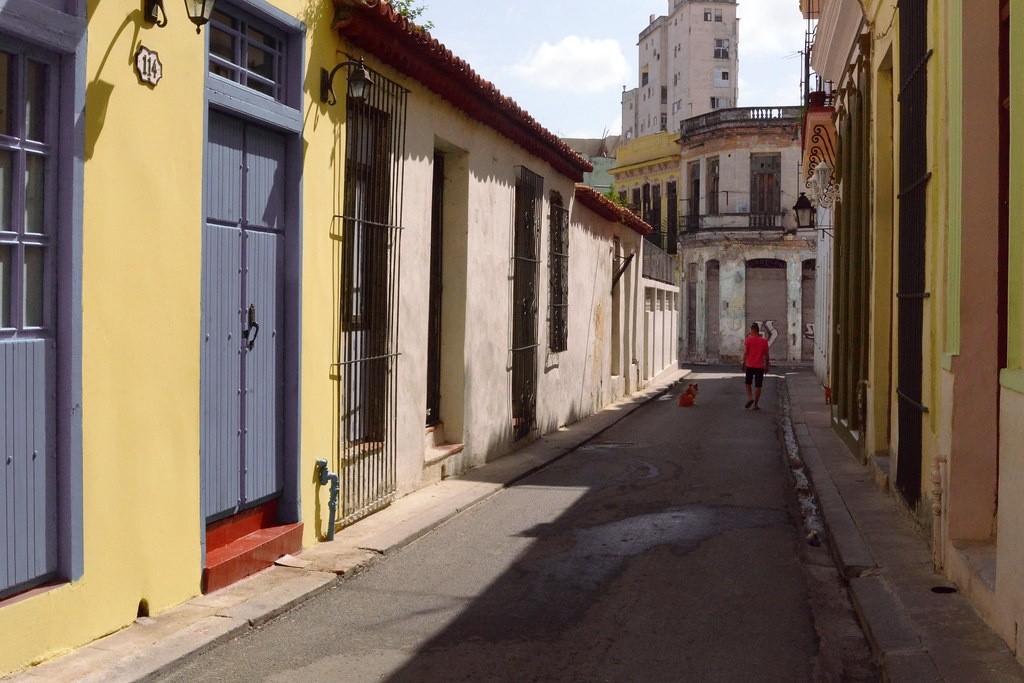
[[805, 158, 844, 213], [326, 56, 371, 108], [792, 190, 819, 233], [183, 0, 216, 35]]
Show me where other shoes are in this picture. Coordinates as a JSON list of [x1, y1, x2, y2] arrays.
[[745, 399, 754, 408], [753, 407, 760, 410]]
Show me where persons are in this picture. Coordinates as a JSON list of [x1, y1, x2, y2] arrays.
[[742, 323, 769, 410]]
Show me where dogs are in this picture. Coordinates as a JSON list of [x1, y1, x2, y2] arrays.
[[678, 384, 700, 405]]
